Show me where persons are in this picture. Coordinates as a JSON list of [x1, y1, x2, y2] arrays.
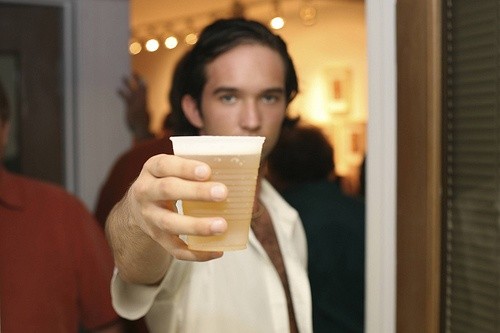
[[113, 68, 160, 138], [107, 16, 314, 332]]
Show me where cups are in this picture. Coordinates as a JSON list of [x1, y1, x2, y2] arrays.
[[169, 136, 266, 252]]
